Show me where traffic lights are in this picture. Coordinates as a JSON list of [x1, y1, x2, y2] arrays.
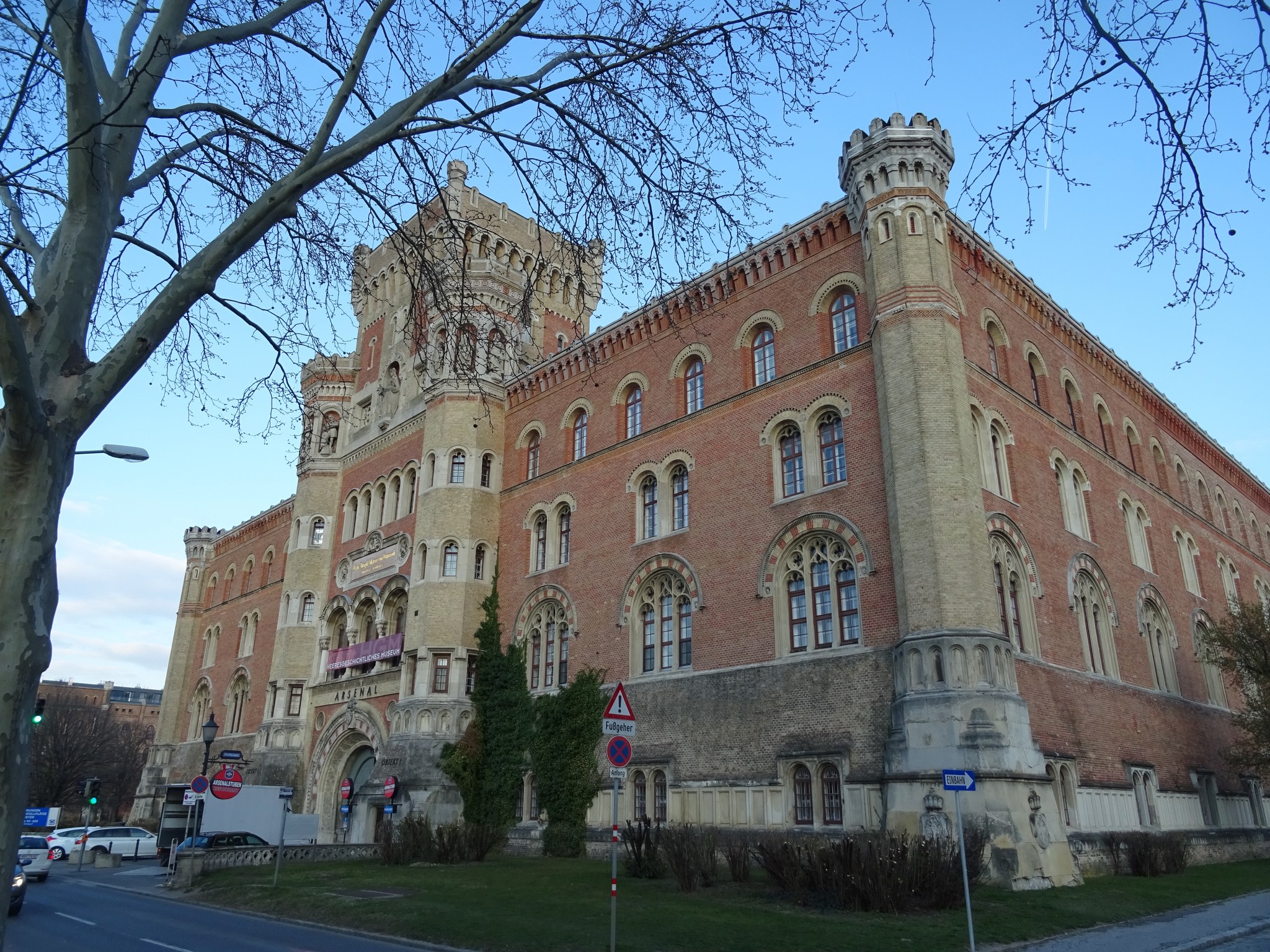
[[33, 699, 46, 724], [76, 779, 101, 805]]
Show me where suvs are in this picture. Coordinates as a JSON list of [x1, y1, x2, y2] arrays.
[[172, 831, 278, 871], [71, 826, 160, 860], [45, 827, 111, 861], [17, 834, 54, 882]]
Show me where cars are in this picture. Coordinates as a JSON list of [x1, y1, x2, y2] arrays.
[[9, 856, 32, 916]]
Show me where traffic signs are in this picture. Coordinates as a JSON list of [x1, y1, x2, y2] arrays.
[[942, 769, 976, 791]]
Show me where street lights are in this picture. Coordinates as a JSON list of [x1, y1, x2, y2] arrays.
[[189, 711, 220, 886]]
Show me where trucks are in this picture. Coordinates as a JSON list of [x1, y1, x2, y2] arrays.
[[154, 783, 293, 849]]
